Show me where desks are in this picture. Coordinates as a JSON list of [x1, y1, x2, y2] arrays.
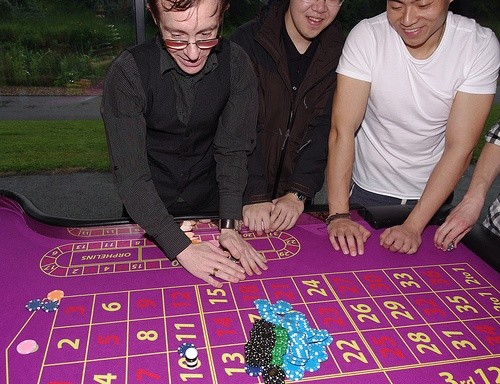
[[0, 188, 500, 384]]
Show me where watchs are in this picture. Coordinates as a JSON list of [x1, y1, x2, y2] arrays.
[[288, 188, 312, 203]]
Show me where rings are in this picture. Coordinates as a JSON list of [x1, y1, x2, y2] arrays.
[[213, 268, 219, 277]]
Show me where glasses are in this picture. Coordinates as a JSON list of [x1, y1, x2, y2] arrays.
[[301, 0, 344, 6], [157, 12, 223, 49]]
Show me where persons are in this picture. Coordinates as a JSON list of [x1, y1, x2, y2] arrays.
[[229, 0, 348, 236], [327, 0, 500, 256], [433, 117, 500, 251], [100, 0, 269, 289]]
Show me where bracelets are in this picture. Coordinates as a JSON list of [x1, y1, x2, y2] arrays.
[[326, 213, 352, 226], [219, 219, 239, 230]]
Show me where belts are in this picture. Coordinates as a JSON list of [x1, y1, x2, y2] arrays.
[[352, 186, 454, 206]]
